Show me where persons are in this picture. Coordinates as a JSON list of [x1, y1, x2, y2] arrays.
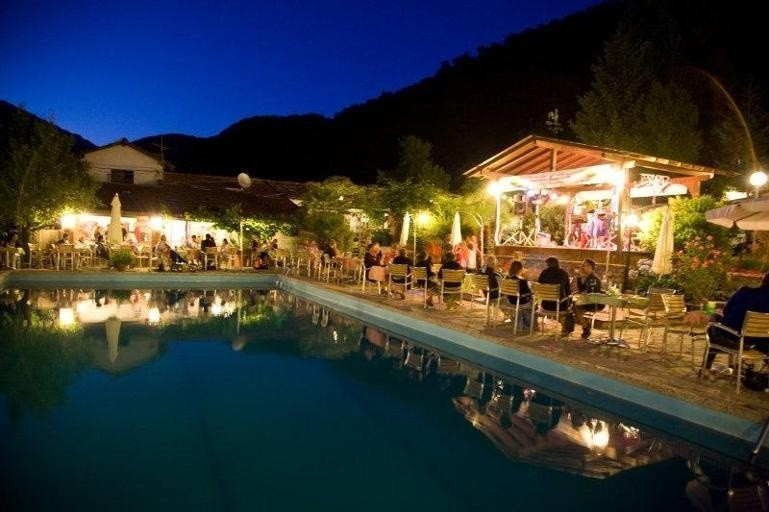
[[94, 289, 106, 307], [480, 254, 507, 300], [154, 287, 269, 313], [698, 271, 769, 379], [94, 225, 282, 274], [503, 260, 538, 331], [571, 258, 607, 340], [3, 231, 22, 271], [2, 287, 17, 311], [500, 379, 523, 429], [55, 232, 70, 246], [359, 323, 383, 362], [474, 371, 494, 415], [528, 390, 563, 447], [536, 256, 574, 338], [317, 236, 478, 312]]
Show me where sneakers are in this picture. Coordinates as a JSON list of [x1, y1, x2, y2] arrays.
[[744, 370, 769, 391]]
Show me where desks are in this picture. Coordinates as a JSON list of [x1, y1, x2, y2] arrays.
[[570, 289, 649, 347]]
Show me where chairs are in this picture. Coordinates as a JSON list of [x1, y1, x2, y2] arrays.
[[1, 239, 706, 359], [386, 336, 765, 510], [695, 310, 768, 394]]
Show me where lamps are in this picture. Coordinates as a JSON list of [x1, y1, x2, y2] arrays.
[[749, 172, 767, 198]]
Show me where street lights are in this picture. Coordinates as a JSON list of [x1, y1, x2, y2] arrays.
[[748, 170, 768, 257]]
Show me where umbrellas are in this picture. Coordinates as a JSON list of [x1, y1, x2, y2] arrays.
[[650, 200, 674, 280], [399, 211, 410, 248], [103, 300, 122, 365], [704, 196, 768, 233], [106, 193, 124, 246], [448, 211, 462, 248]]
